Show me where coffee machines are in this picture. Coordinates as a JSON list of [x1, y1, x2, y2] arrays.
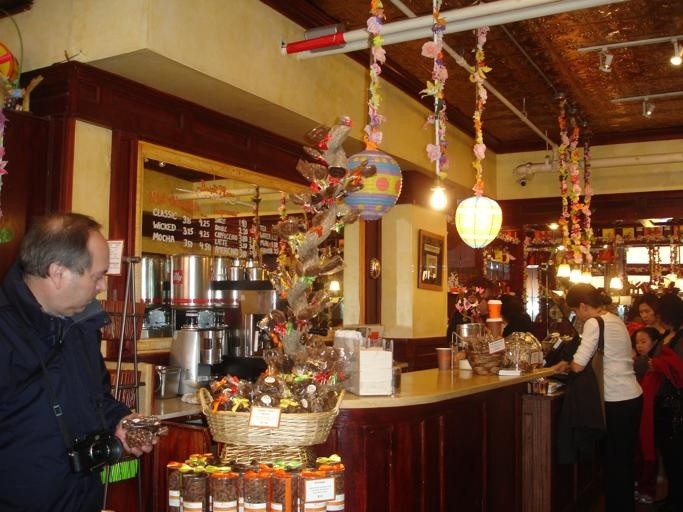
[[169, 311, 229, 398]]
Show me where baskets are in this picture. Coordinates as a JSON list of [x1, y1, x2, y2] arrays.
[[199, 388, 346, 445], [218, 444, 316, 471]]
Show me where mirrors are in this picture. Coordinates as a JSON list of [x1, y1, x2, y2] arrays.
[[132, 140, 364, 360]]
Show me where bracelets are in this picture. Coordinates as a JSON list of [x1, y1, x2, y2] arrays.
[[566, 359, 572, 372]]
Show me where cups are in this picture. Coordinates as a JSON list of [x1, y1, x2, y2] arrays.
[[487, 299, 503, 318], [436, 349, 452, 371]]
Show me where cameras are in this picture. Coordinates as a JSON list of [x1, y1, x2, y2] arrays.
[[69, 433, 112, 474]]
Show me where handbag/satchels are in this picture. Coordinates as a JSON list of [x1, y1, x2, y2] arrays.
[[592, 350, 606, 425]]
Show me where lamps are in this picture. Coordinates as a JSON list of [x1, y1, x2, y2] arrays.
[[572, 33, 682, 120], [522, 247, 542, 323], [452, 20, 502, 251], [547, 95, 627, 293], [420, 3, 451, 210], [340, 1, 403, 223]]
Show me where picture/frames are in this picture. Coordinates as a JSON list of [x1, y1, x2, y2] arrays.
[[415, 228, 446, 294]]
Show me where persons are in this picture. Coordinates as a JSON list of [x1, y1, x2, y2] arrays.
[[550, 278, 646, 512], [623, 289, 683, 505], [2, 206, 162, 512], [438, 278, 532, 342]]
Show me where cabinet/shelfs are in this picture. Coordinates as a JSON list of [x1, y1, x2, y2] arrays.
[[100, 253, 142, 510], [521, 382, 571, 512]]
